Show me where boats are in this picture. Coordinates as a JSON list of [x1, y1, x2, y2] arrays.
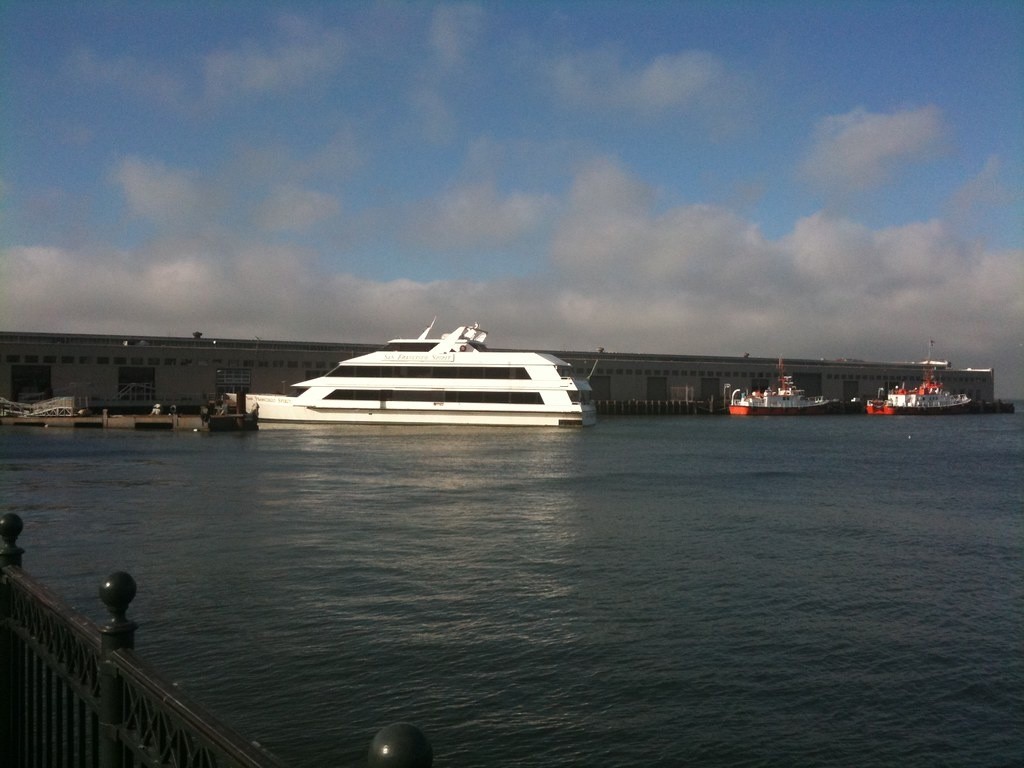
[[729, 356, 830, 417], [221, 316, 601, 430], [865, 367, 972, 416]]
